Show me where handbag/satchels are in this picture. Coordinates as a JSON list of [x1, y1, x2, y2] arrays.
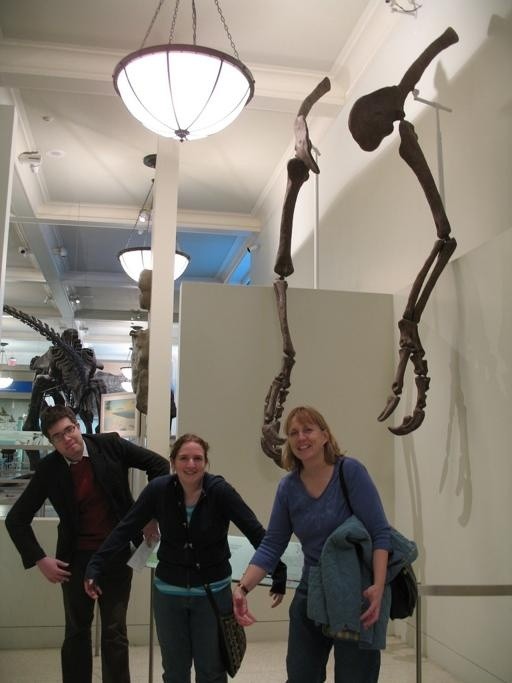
[[388, 560, 420, 622], [217, 612, 248, 678]]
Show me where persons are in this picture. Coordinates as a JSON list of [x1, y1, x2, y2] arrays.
[[83, 434, 287, 683], [232, 406, 393, 683], [5, 405, 170, 683]]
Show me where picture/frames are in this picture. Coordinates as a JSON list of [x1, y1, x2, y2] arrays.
[[98, 388, 141, 438]]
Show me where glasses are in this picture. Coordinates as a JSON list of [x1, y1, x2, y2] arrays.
[[48, 423, 78, 445]]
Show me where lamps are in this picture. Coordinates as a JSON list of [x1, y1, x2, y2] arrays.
[[110, 0, 257, 146], [121, 370, 135, 392], [113, 152, 193, 289], [119, 346, 135, 381]]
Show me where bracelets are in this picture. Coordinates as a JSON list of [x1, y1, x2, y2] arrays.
[[238, 583, 248, 594]]
